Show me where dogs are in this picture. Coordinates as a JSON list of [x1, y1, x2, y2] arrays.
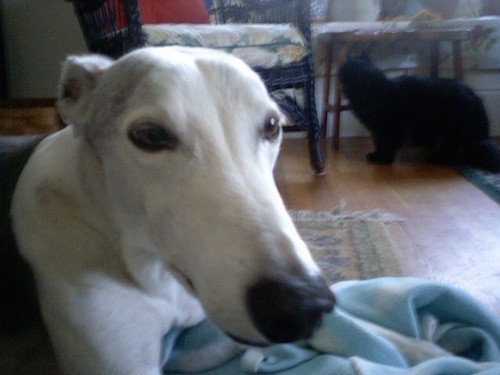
[[12, 45, 337, 375]]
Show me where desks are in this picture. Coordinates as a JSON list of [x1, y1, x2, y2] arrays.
[[307, 16, 500, 157]]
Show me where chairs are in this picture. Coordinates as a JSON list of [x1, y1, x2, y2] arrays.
[[71, 1, 330, 171]]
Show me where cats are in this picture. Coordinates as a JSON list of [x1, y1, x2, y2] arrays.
[[336, 46, 500, 171]]
